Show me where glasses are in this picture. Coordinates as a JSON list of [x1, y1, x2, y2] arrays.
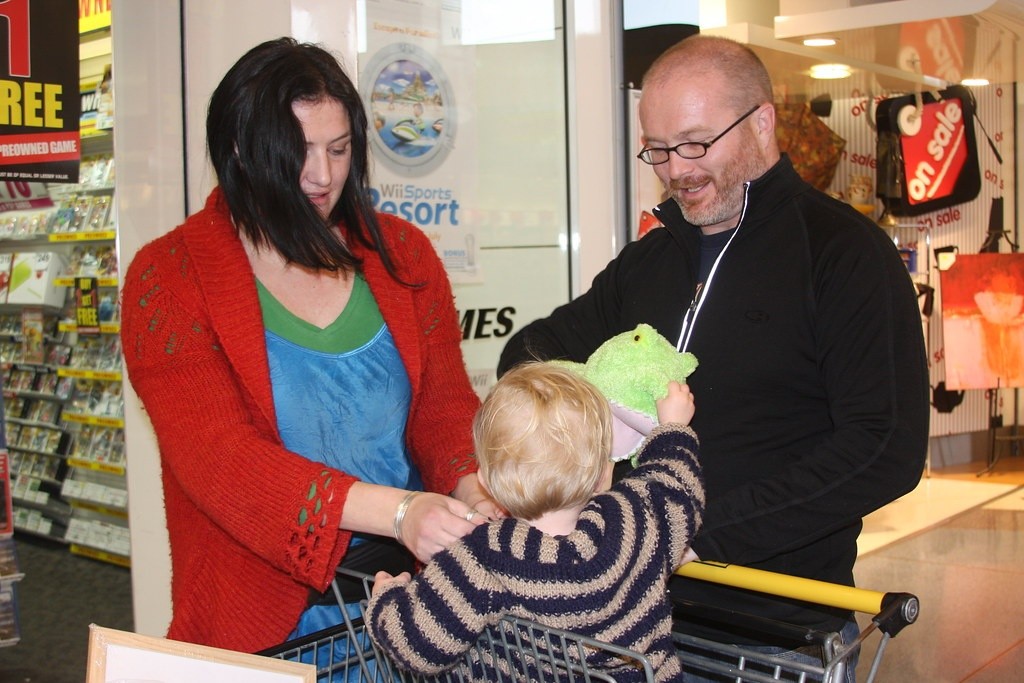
[[637, 105, 761, 165]]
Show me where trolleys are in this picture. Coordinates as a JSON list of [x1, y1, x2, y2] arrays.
[[241, 541, 921, 683]]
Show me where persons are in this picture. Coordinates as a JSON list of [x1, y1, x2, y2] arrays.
[[498, 38, 929, 682], [122, 38, 511, 683], [363, 361, 705, 683]]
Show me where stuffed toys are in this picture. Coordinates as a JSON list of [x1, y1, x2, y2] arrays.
[[548, 324, 699, 468]]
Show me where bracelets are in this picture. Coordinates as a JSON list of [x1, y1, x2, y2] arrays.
[[394, 490, 421, 545]]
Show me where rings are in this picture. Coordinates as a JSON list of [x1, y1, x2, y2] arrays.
[[466, 508, 478, 520]]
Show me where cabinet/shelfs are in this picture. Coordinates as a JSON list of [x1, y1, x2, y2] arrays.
[[0, 188, 128, 573]]
